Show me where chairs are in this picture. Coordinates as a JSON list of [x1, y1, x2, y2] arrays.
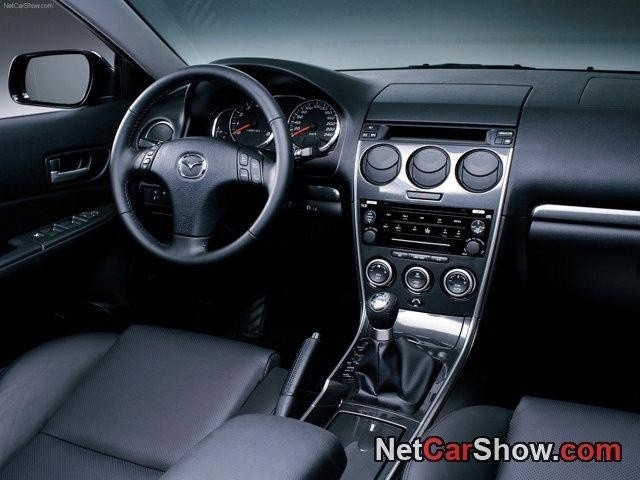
[[401, 396, 638, 480], [0, 324, 347, 480]]
